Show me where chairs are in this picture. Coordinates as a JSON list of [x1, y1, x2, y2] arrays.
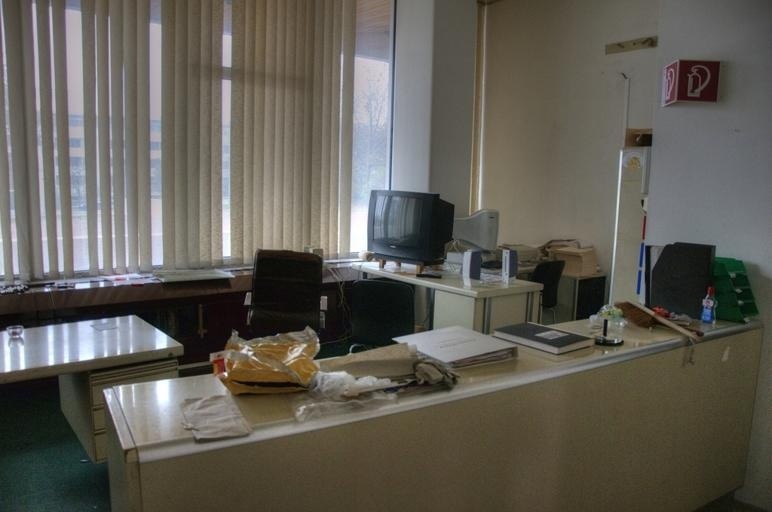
[[346, 278, 416, 354], [243, 247, 328, 340], [528, 258, 567, 324]]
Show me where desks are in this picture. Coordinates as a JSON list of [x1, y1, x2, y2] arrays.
[[492, 262, 610, 323], [103, 306, 766, 512], [348, 259, 545, 336], [0, 311, 186, 468], [2, 257, 359, 317]]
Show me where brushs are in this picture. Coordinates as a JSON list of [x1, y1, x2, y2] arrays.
[[614, 299, 697, 340]]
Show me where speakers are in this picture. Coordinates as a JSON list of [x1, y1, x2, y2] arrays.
[[501, 249, 519, 284], [463, 249, 481, 288]]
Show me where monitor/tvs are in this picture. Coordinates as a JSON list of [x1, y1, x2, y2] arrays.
[[452, 209, 499, 252], [367, 189, 455, 266]]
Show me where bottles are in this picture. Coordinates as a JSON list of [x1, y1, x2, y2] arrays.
[[699, 286, 718, 324]]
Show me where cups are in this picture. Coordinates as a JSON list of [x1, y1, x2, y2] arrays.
[[8, 337, 25, 348], [7, 325, 24, 338]]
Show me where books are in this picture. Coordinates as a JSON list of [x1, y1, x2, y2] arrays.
[[491, 321, 598, 355], [495, 237, 599, 279]]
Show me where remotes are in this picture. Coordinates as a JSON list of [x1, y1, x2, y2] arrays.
[[416, 272, 442, 279]]
